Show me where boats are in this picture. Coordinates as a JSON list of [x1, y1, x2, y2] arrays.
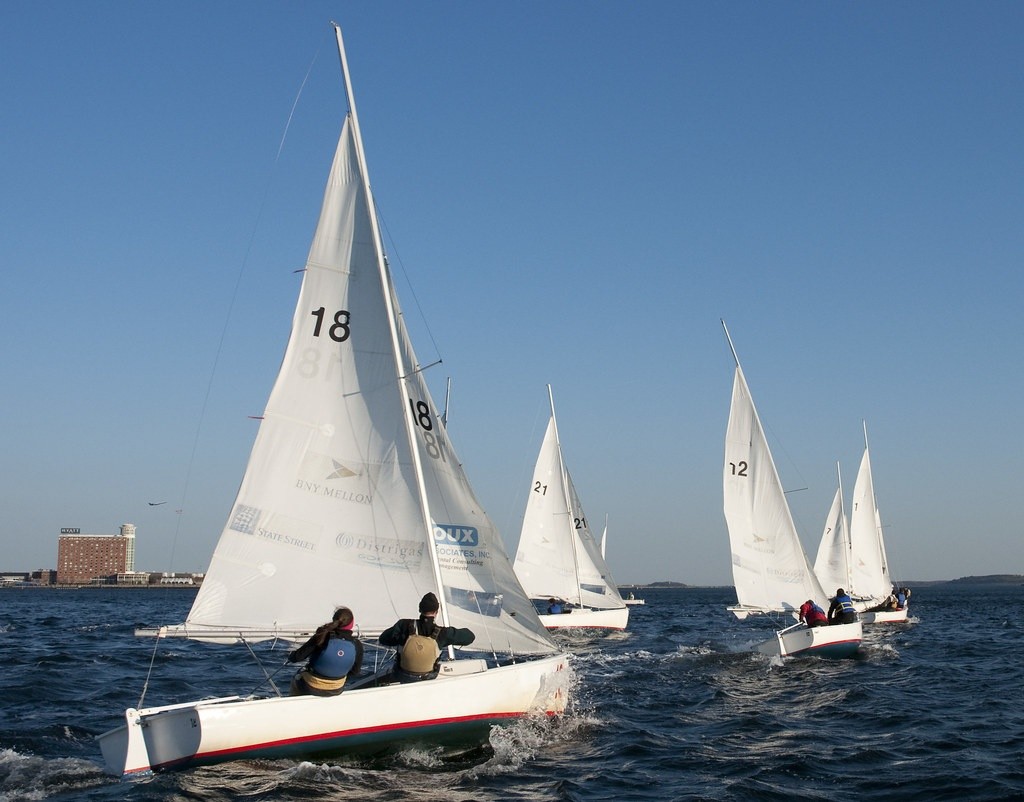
[[97, 694, 244, 778]]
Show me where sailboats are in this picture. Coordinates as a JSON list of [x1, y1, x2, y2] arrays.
[[716, 316, 863, 659], [510, 383, 628, 630], [135, 19, 577, 773], [851, 419, 910, 625], [600, 512, 644, 606]]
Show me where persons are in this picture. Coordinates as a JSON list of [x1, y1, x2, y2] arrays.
[[626, 591, 635, 600], [380, 591, 478, 683], [547, 598, 563, 631], [880, 587, 912, 612], [798, 599, 829, 629], [286, 607, 365, 697], [828, 588, 856, 625]]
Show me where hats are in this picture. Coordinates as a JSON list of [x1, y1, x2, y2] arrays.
[[419, 592, 439, 611]]
